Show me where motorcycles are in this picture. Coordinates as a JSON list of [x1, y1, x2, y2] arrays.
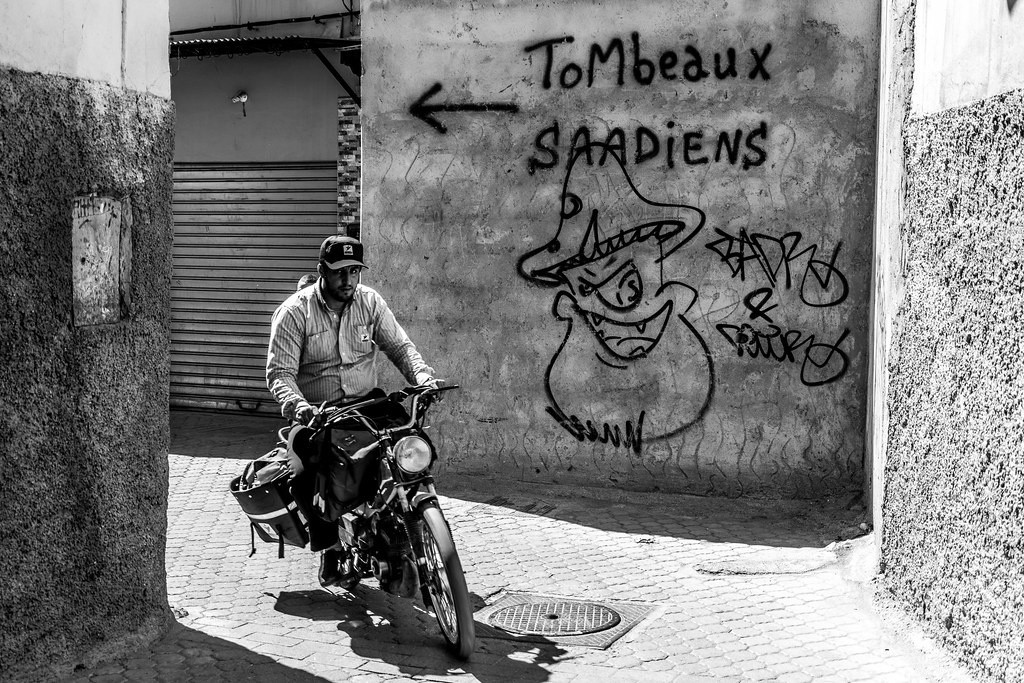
[[277, 382, 475, 660]]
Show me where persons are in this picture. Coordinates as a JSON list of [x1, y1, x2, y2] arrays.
[[265, 233, 447, 588]]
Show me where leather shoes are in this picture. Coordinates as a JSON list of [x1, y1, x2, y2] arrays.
[[318, 546, 347, 587]]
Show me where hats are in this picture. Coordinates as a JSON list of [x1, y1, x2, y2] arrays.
[[318, 234, 369, 270]]
